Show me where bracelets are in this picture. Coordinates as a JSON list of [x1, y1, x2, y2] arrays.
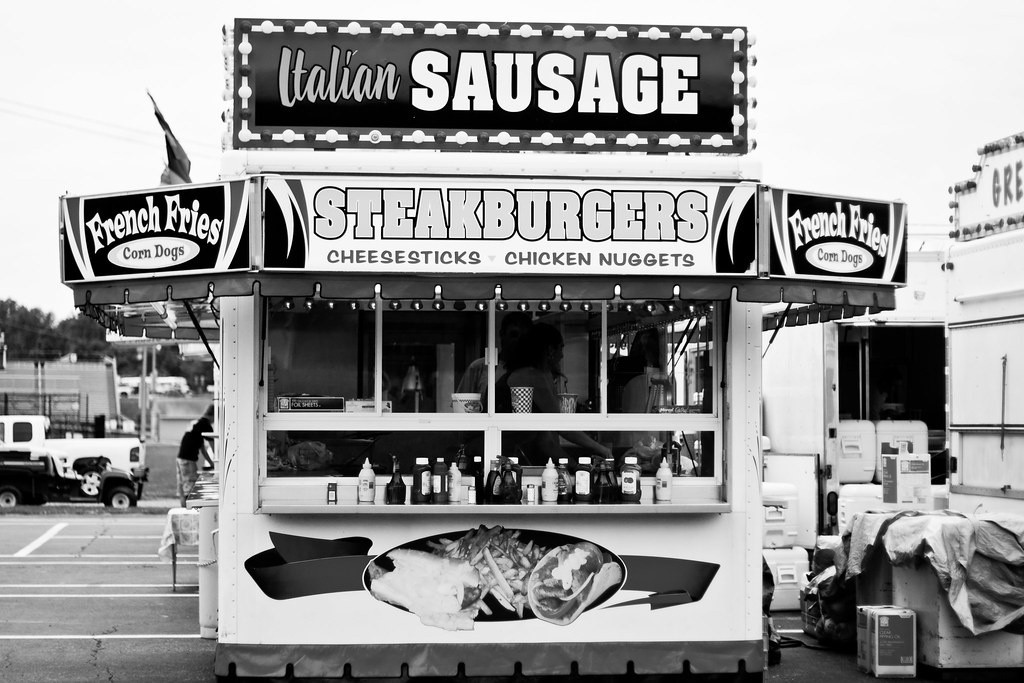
[[575, 464, 580, 472]]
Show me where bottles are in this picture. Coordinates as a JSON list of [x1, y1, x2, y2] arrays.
[[384, 455, 406, 504], [400, 358, 424, 412], [486, 458, 501, 504], [622, 456, 642, 502], [413, 457, 431, 504], [540, 456, 573, 504], [327, 477, 337, 505], [468, 486, 476, 504], [503, 455, 523, 504], [473, 455, 485, 504], [575, 456, 621, 503], [448, 462, 461, 502], [431, 457, 449, 504], [526, 484, 536, 502], [357, 458, 375, 502], [655, 456, 672, 501]]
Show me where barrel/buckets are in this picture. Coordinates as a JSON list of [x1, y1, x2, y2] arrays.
[[449, 392, 483, 413]]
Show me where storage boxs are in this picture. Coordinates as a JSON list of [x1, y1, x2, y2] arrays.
[[881, 453, 931, 510], [857, 605, 900, 672], [869, 609, 917, 677]]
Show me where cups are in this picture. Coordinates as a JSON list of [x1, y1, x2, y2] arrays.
[[556, 393, 579, 413], [510, 386, 533, 412]]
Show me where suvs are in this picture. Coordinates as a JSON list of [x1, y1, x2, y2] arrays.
[[0, 412, 150, 511]]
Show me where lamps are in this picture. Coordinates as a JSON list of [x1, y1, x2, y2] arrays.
[[161, 308, 177, 331], [600, 331, 636, 368], [150, 301, 166, 316]]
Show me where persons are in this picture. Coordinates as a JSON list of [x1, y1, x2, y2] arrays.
[[455, 311, 533, 407], [607, 328, 660, 469], [177, 404, 214, 509], [480, 323, 613, 472]]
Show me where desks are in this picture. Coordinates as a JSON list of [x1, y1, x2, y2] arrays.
[[158, 507, 199, 593]]
